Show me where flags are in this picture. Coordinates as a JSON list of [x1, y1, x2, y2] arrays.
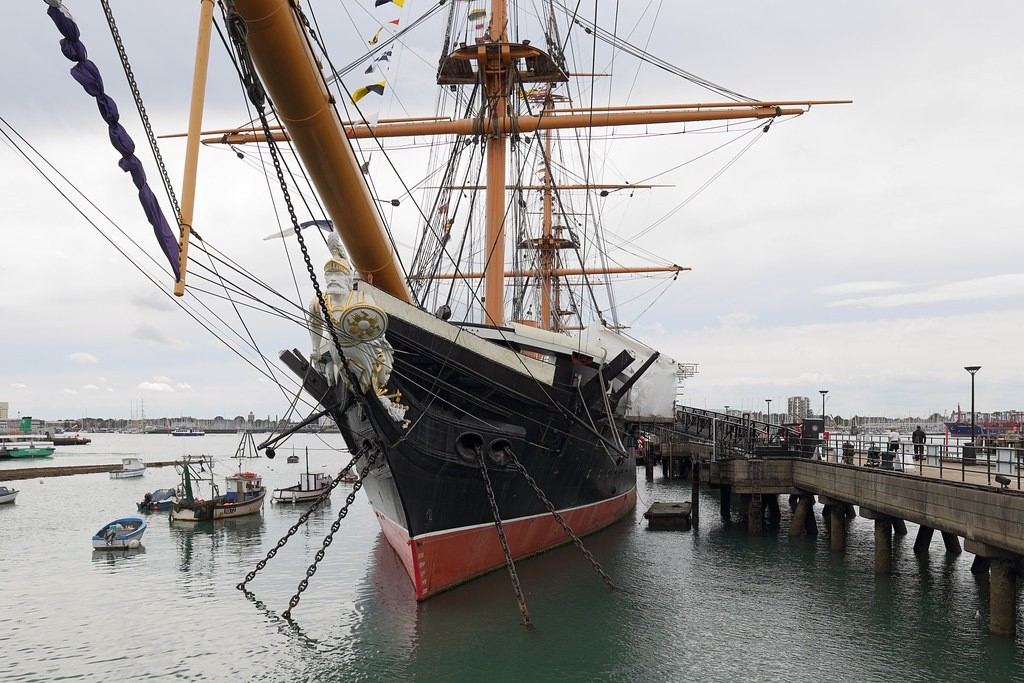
[[364, 44, 394, 74], [468, 9, 486, 21], [368, 18, 399, 45], [476, 24, 483, 29], [439, 203, 448, 214], [375, 0, 405, 8], [351, 81, 385, 104]]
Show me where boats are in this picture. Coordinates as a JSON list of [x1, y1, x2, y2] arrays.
[[944, 403, 1024, 436], [3, 1, 852, 631]]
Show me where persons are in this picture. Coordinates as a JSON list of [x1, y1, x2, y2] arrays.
[[912, 426, 926, 461], [309, 256, 392, 397], [889, 425, 901, 451]]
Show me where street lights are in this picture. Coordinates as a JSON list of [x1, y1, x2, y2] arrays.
[[964, 365, 981, 445]]
